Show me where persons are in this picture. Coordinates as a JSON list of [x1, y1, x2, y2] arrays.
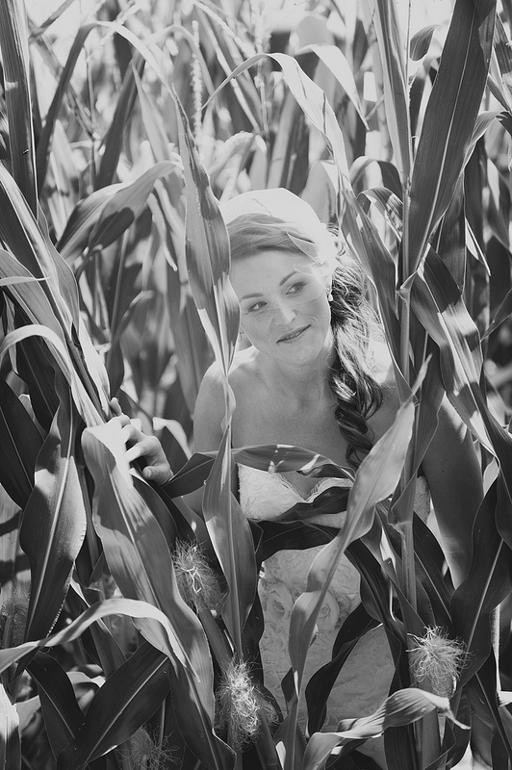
[[104, 187, 431, 769]]
